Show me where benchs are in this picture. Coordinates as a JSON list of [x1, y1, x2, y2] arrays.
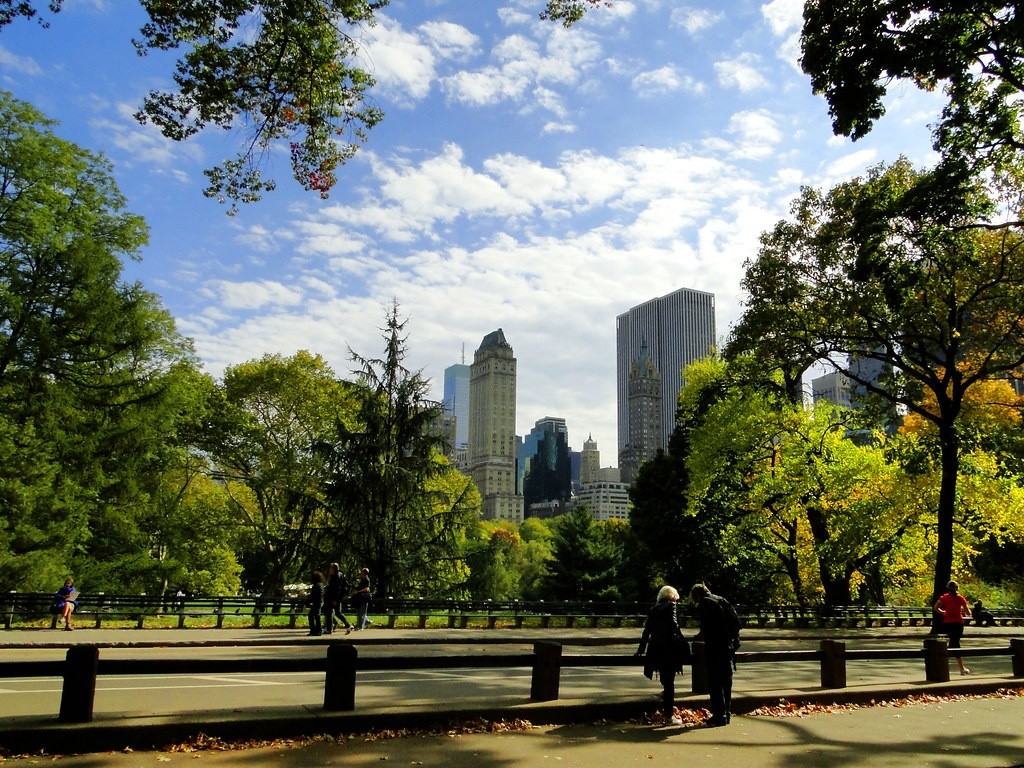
[[3, 590, 1024, 629]]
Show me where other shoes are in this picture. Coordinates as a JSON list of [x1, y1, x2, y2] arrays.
[[665, 715, 683, 726], [705, 712, 731, 727], [961, 667, 970, 675]]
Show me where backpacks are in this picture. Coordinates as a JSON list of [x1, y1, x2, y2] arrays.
[[705, 595, 742, 638]]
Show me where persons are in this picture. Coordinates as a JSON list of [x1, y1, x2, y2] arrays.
[[635, 586, 690, 718], [54, 579, 79, 630], [691, 584, 741, 726], [307, 563, 372, 635], [935, 581, 972, 675]]
